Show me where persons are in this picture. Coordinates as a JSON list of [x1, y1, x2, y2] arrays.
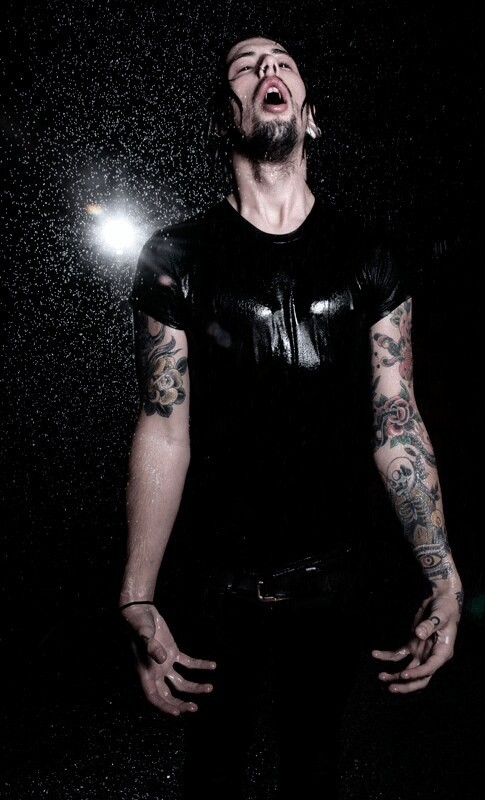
[[116, 34, 465, 800]]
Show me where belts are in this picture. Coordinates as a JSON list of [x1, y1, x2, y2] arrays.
[[192, 532, 389, 602]]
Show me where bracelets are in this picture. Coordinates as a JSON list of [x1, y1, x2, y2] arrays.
[[116, 600, 154, 612]]
